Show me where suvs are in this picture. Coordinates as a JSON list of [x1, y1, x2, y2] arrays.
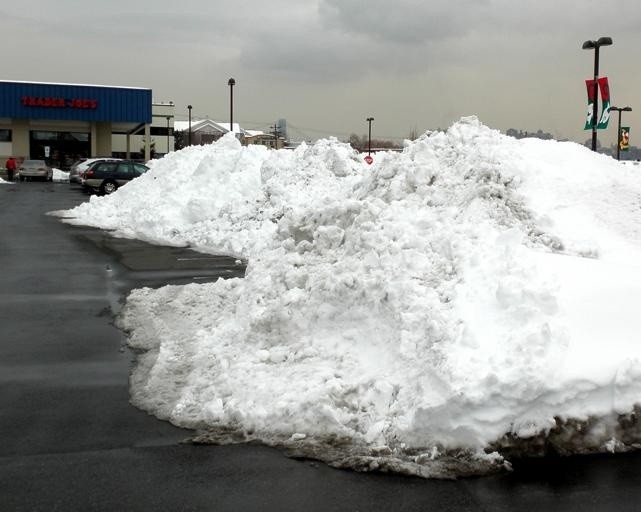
[[81, 161, 151, 196], [69, 158, 125, 190]]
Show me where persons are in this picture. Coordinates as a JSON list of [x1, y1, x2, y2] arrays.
[[6, 156, 16, 181]]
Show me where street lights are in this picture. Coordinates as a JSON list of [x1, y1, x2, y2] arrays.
[[188, 104, 192, 146], [228, 77, 235, 132], [366, 117, 375, 157], [582, 37, 613, 152], [610, 106, 632, 161], [165, 116, 170, 154]]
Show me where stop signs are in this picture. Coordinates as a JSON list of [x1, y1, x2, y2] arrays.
[[363, 156, 373, 165]]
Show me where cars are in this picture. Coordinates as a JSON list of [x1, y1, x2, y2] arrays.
[[18, 160, 53, 182]]
